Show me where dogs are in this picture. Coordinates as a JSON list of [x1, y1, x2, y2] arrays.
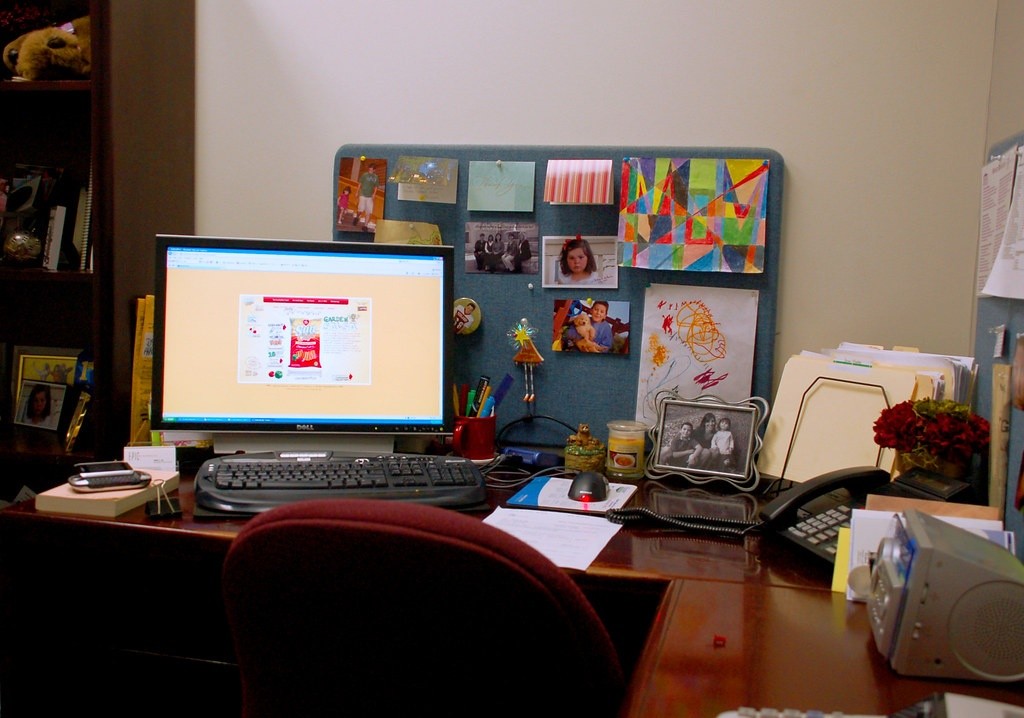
[[573, 314, 609, 353]]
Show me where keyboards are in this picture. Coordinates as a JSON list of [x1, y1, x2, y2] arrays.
[[193, 450, 486, 515]]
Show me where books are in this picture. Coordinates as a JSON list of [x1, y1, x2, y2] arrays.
[[36, 471, 180, 517], [41, 149, 96, 274]]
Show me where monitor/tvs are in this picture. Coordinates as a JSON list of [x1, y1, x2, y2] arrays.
[[152, 231, 455, 458]]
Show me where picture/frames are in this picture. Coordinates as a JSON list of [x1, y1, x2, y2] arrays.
[[13, 380, 69, 431], [642, 388, 769, 491], [10, 347, 83, 418], [42, 206, 67, 270]]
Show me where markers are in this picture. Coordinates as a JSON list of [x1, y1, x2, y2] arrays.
[[495, 374, 514, 414], [468, 374, 490, 417]]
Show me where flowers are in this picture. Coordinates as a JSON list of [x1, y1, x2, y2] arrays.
[[873, 400, 992, 469]]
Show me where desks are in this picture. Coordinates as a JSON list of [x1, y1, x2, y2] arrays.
[[624, 578, 1024, 718], [1, 463, 833, 588]]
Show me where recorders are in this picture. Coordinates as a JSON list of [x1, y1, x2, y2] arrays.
[[866, 508, 1024, 684]]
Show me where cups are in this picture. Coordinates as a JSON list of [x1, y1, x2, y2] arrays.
[[453, 414, 497, 459], [607, 421, 645, 475]]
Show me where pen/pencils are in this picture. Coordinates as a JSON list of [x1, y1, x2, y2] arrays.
[[452, 383, 476, 417], [480, 396, 495, 418], [477, 386, 491, 418]]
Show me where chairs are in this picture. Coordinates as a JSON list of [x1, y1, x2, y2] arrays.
[[222, 499, 627, 718]]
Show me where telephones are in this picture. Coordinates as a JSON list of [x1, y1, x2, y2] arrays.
[[760, 465, 970, 580]]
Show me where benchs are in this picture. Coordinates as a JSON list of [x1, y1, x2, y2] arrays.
[[484, 256, 538, 274]]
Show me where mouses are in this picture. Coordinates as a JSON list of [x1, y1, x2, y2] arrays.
[[568, 471, 612, 503]]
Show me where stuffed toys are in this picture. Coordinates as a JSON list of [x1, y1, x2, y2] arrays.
[[3, 17, 91, 80]]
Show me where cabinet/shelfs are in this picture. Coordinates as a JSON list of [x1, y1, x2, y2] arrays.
[[0, 0, 116, 464]]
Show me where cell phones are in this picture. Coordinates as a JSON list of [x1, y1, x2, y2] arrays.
[[68, 461, 152, 492]]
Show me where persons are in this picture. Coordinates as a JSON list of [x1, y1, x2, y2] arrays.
[[24, 384, 53, 428], [353, 163, 379, 231], [662, 412, 736, 471], [454, 303, 475, 334], [558, 238, 600, 285], [567, 301, 612, 353], [40, 364, 67, 383], [337, 186, 352, 224], [474, 232, 532, 273]]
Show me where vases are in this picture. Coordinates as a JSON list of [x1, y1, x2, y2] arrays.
[[896, 450, 969, 478]]
